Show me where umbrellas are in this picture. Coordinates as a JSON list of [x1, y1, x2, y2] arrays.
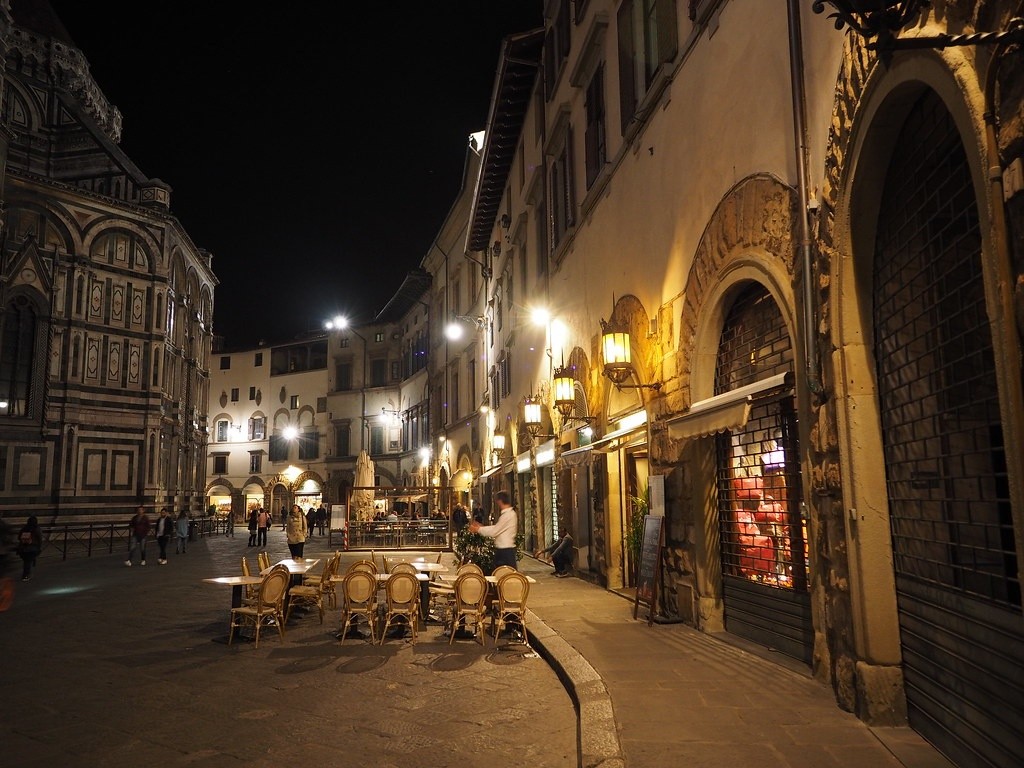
[[350, 450, 374, 545]]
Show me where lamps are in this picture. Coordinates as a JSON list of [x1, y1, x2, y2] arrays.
[[598, 290, 661, 393], [523, 380, 557, 440], [552, 347, 597, 424], [491, 418, 516, 462]]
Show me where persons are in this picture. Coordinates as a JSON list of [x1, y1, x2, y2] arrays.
[[248, 508, 272, 547], [316, 504, 327, 535], [207, 504, 216, 522], [225, 511, 235, 537], [432, 508, 446, 519], [281, 506, 288, 531], [16, 516, 43, 582], [123, 506, 150, 567], [403, 509, 421, 520], [155, 507, 173, 565], [373, 512, 385, 521], [535, 526, 573, 577], [469, 490, 518, 571], [387, 511, 397, 521], [175, 510, 189, 553], [306, 508, 316, 537], [285, 505, 308, 558], [453, 502, 484, 537]]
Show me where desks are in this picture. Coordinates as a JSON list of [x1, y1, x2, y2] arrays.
[[415, 566, 449, 620], [199, 576, 265, 646], [279, 559, 320, 612], [330, 573, 430, 636], [409, 563, 443, 576], [274, 562, 318, 618], [438, 575, 536, 637], [260, 565, 310, 625]]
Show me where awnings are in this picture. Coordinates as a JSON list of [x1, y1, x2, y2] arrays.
[[560, 428, 647, 471], [479, 465, 502, 483], [396, 494, 428, 503], [666, 395, 753, 445]]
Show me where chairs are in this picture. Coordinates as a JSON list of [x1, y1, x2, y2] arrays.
[[227, 549, 530, 649], [349, 515, 447, 547]]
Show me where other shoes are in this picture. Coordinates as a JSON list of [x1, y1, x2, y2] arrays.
[[21, 577, 31, 582], [551, 571, 558, 575], [158, 558, 163, 563], [141, 560, 146, 566], [556, 572, 568, 577], [160, 560, 167, 565], [124, 560, 131, 566]]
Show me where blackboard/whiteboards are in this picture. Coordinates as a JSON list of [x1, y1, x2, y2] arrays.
[[330, 504, 347, 532], [636, 516, 667, 604]]
